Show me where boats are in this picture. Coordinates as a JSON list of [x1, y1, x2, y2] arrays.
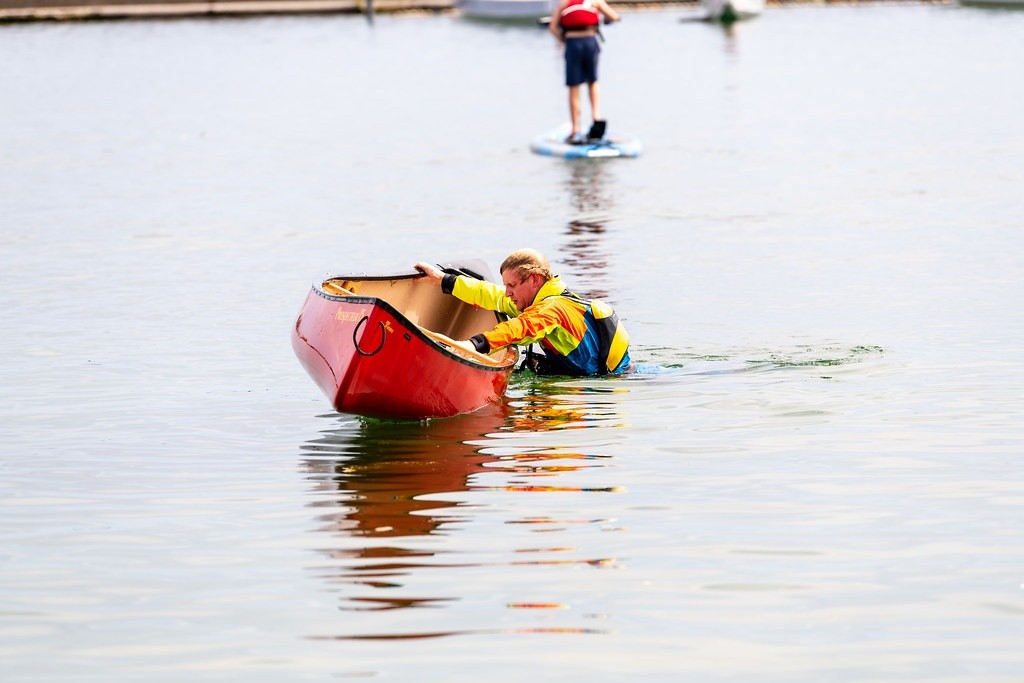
[[287, 257, 522, 425]]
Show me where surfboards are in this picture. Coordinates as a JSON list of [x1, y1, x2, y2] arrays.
[[529, 132, 642, 159]]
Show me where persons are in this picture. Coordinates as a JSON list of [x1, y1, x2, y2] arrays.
[[549, 0, 621, 144], [413, 250, 638, 374]]
